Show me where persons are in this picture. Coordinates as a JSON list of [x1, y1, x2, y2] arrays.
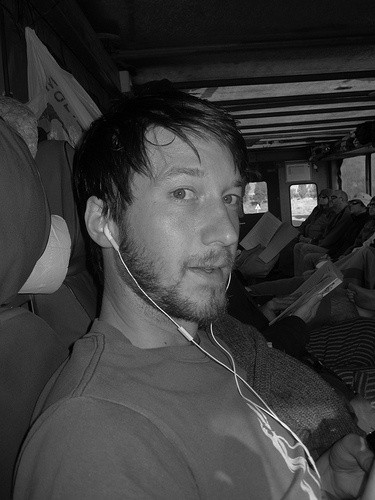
[[226, 240, 374, 403], [298, 189, 375, 262], [12, 79, 375, 500]]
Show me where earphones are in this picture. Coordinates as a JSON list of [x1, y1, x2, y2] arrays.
[[103, 223, 120, 250]]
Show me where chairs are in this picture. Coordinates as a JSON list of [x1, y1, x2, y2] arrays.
[[0, 119, 71, 500], [34, 140, 102, 349]]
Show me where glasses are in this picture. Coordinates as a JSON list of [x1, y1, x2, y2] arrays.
[[370, 202, 375, 206], [319, 195, 326, 199], [331, 196, 342, 200], [348, 201, 362, 205]]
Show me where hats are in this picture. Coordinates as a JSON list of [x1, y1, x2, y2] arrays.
[[348, 192, 372, 208], [318, 189, 332, 200]]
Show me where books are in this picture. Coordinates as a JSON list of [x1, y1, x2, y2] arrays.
[[239, 212, 301, 263], [269, 260, 342, 325]]
